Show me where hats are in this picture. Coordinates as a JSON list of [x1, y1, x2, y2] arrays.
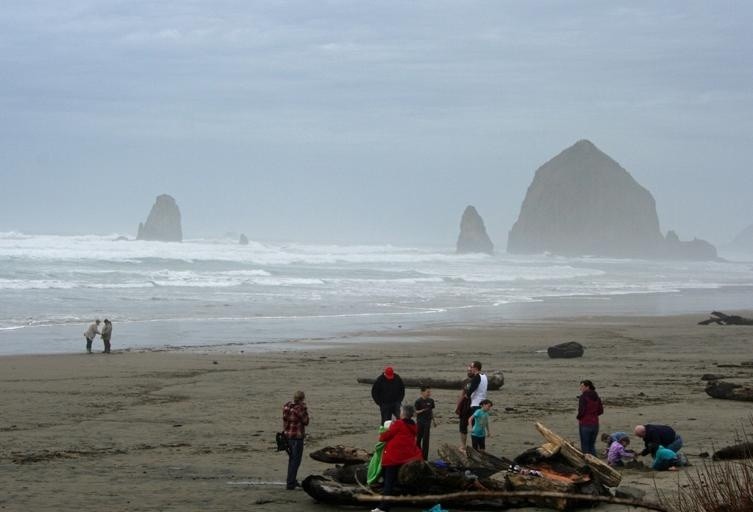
[[385, 366, 394, 380]]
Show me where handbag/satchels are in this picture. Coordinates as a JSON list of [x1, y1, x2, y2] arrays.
[[276, 431, 290, 451]]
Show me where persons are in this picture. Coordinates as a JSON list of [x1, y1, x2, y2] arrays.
[[576, 379, 604, 462], [277, 388, 310, 487], [372, 363, 491, 465], [601, 425, 688, 472], [100, 319, 113, 352], [83, 319, 102, 353]]
